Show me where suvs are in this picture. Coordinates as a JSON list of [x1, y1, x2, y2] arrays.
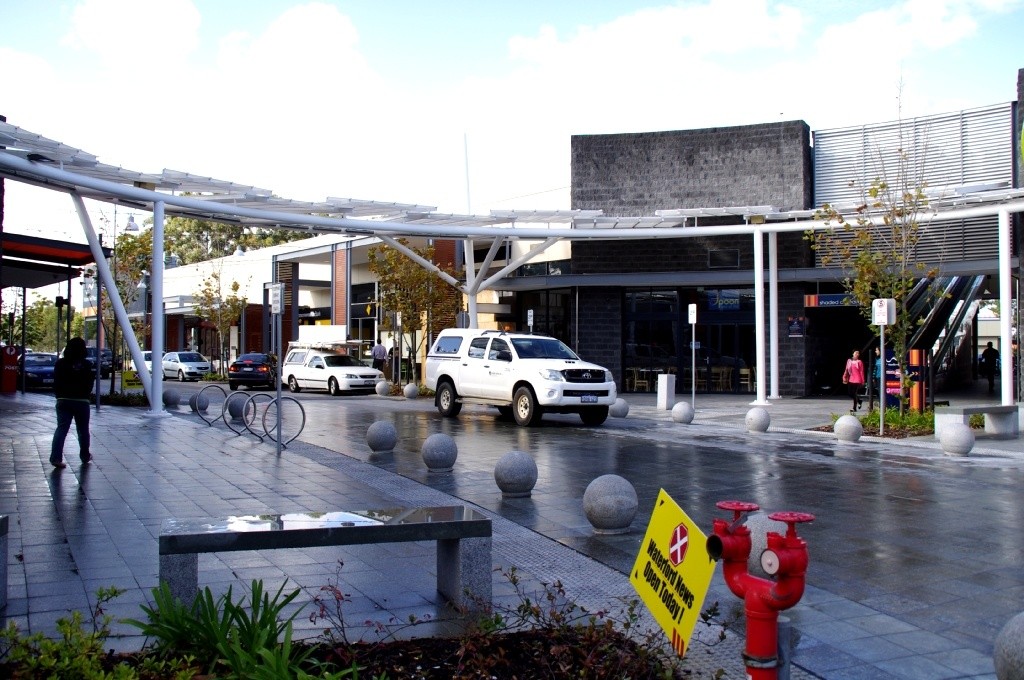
[[425, 328, 617, 428], [281, 346, 386, 397]]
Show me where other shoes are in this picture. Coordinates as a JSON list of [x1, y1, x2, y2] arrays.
[[87, 454, 93, 461], [52, 460, 66, 469]]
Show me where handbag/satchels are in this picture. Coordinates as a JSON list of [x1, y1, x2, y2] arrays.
[[70, 375, 91, 398], [843, 373, 849, 384]]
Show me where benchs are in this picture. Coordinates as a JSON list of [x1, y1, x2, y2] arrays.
[[158, 506, 493, 606], [934, 404, 1019, 438]]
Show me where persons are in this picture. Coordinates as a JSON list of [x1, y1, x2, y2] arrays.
[[872, 346, 881, 396], [843, 348, 865, 411], [532, 341, 543, 356], [372, 339, 389, 371], [49, 337, 94, 468], [978, 341, 999, 391], [388, 340, 403, 384], [503, 341, 521, 357]]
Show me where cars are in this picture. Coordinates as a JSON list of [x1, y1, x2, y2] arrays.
[[130, 350, 168, 375], [1, 341, 122, 391], [161, 351, 212, 382], [228, 353, 283, 390]]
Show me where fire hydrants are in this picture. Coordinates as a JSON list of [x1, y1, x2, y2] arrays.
[[705, 500, 816, 680]]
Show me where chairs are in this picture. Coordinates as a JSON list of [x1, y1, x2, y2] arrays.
[[655, 367, 671, 392], [634, 367, 648, 392], [695, 366, 757, 392]]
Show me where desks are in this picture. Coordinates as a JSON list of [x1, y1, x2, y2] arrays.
[[626, 368, 663, 379]]
